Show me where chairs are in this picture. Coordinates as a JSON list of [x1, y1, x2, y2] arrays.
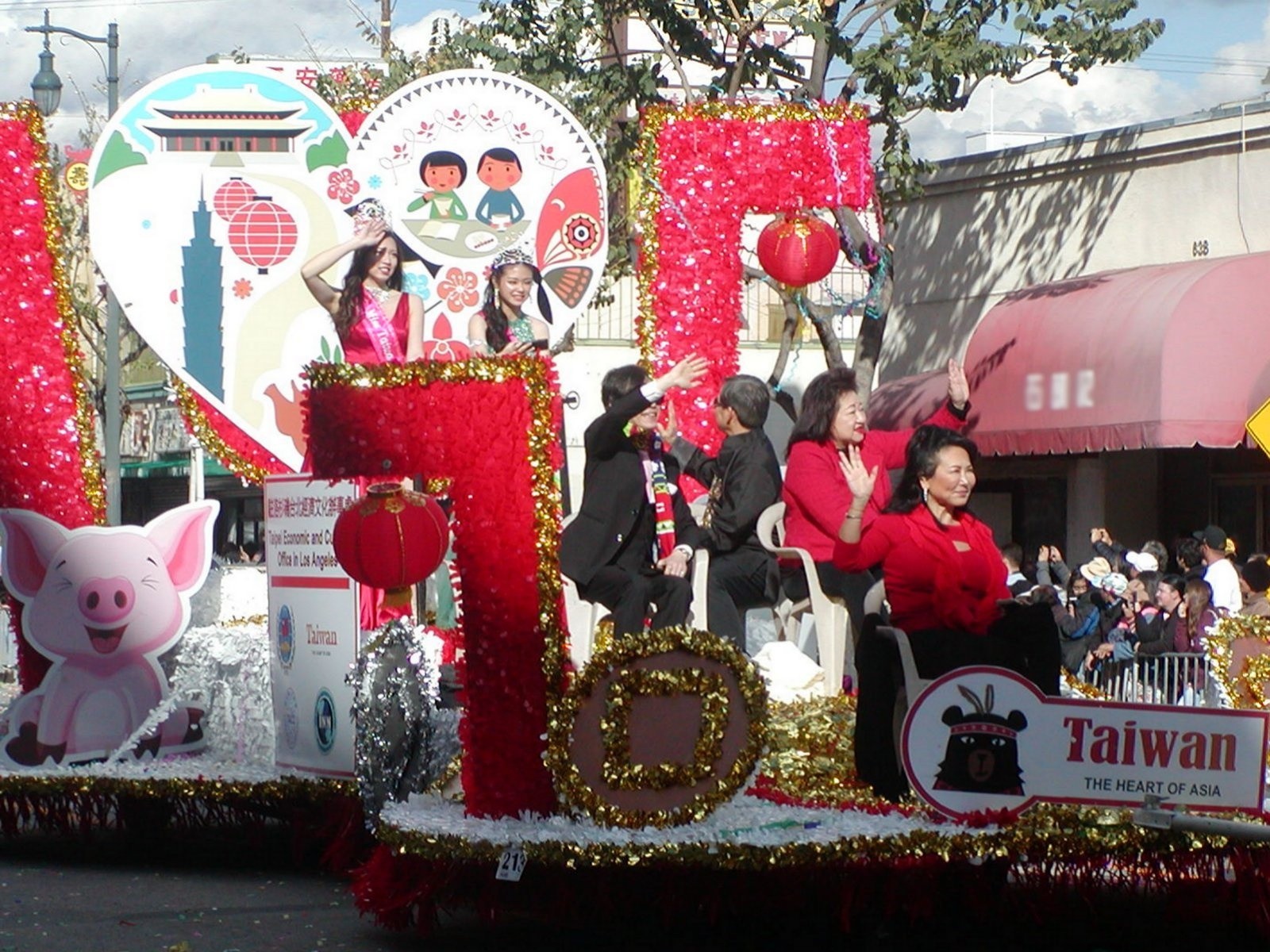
[[756, 501, 849, 695], [863, 575, 937, 773], [556, 513, 709, 678]]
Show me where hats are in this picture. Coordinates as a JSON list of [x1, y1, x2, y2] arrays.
[[1079, 556, 1111, 581], [1092, 572, 1129, 595], [1121, 579, 1149, 602], [1224, 538, 1235, 556], [1193, 525, 1227, 551], [1126, 550, 1158, 572]]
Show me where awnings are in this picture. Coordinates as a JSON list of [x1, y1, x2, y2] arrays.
[[866, 369, 950, 431], [963, 251, 1270, 456]]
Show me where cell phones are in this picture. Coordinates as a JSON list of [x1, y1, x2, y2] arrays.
[[523, 339, 549, 350]]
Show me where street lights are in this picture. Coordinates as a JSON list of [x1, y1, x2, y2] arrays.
[[20, 9, 123, 527]]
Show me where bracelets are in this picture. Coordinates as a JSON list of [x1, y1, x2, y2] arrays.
[[845, 512, 862, 518]]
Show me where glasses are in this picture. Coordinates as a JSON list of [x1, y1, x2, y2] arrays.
[[713, 396, 734, 408]]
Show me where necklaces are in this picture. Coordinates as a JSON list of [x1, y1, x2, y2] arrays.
[[365, 286, 390, 303]]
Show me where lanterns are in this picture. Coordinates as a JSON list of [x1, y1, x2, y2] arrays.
[[757, 214, 840, 296], [332, 483, 449, 608]]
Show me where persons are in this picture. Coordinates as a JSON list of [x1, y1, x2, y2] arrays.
[[301, 219, 425, 363], [1225, 538, 1270, 620], [778, 357, 971, 803], [559, 352, 711, 649], [1003, 528, 1186, 704], [832, 423, 1062, 696], [468, 247, 550, 358], [240, 528, 265, 564], [1176, 525, 1242, 612], [221, 542, 244, 563], [1174, 579, 1221, 704], [656, 375, 783, 651]]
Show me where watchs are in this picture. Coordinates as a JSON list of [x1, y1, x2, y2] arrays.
[[679, 548, 689, 558]]
